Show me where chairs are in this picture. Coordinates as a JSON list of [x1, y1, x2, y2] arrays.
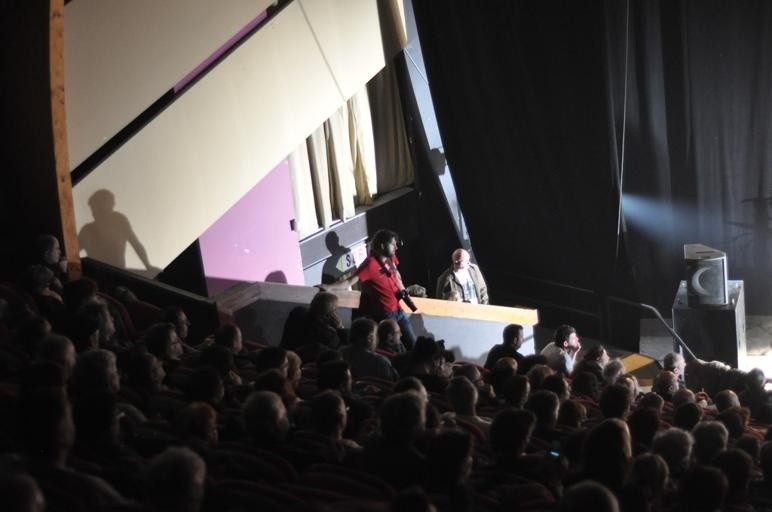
[[0, 285, 771, 512]]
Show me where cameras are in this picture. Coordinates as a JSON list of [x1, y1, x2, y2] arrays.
[[397, 290, 417, 312]]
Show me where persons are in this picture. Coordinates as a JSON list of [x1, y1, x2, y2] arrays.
[[405, 284, 427, 298], [241, 292, 642, 511], [588, 352, 771, 511], [436, 248, 489, 304], [1, 233, 250, 510], [317, 231, 416, 352]]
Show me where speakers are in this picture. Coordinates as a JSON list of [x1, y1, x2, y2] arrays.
[[684, 243, 729, 307], [672, 280, 746, 372]]
